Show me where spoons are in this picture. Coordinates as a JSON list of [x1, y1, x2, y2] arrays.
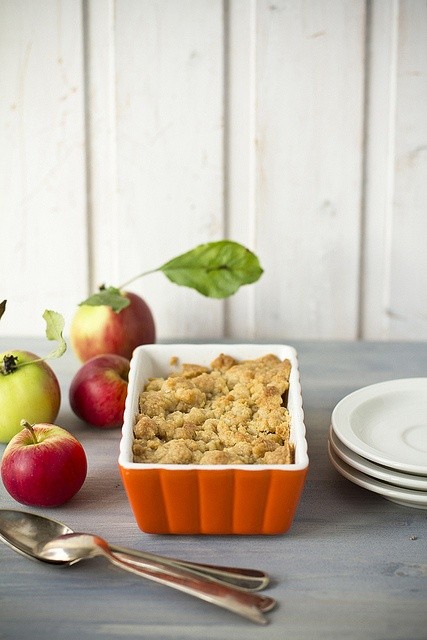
[[0, 509, 270, 594], [32, 533, 275, 625]]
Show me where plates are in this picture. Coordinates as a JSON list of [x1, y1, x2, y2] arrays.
[[326, 378, 427, 511]]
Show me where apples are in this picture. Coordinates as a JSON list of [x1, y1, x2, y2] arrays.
[[70, 291, 155, 364], [69, 354, 130, 428], [0, 350, 61, 444], [1, 422, 87, 507]]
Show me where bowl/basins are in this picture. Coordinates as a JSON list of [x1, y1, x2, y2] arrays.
[[117, 343, 309, 535]]
